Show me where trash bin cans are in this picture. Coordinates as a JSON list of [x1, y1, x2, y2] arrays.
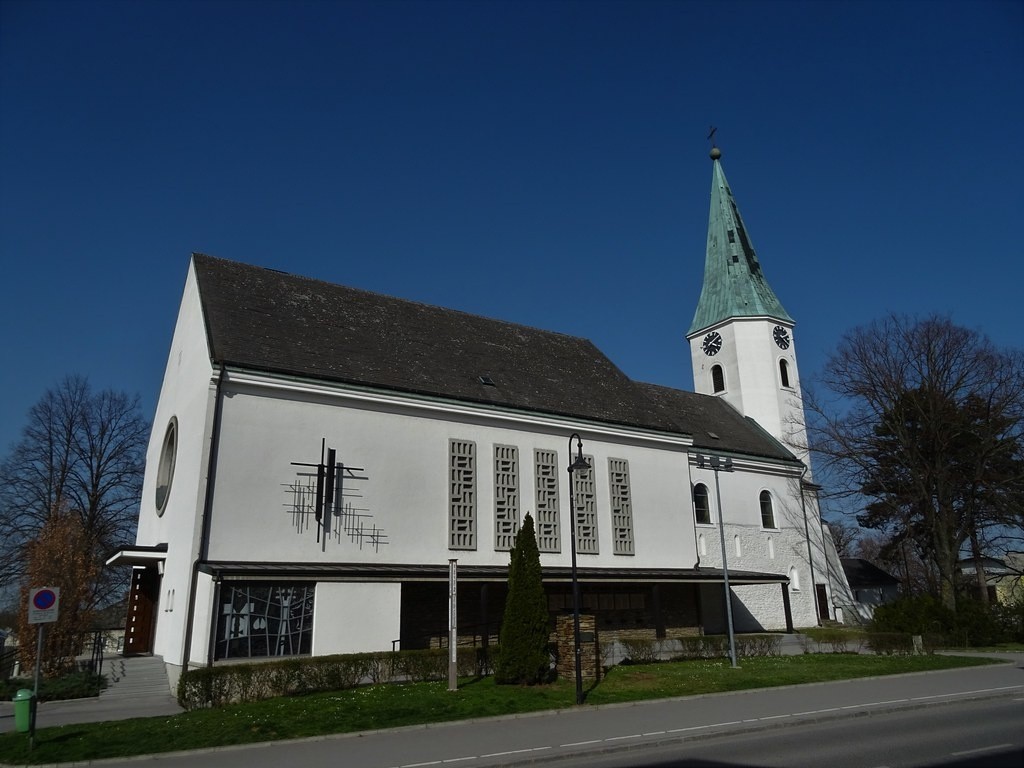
[[11, 689, 34, 733]]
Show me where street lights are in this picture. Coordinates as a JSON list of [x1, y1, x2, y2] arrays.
[[694, 453, 742, 668], [893, 526, 912, 600], [567, 433, 592, 705]]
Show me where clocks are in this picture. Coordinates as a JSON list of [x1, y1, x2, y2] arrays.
[[773, 326, 790, 350], [703, 332, 722, 356]]
